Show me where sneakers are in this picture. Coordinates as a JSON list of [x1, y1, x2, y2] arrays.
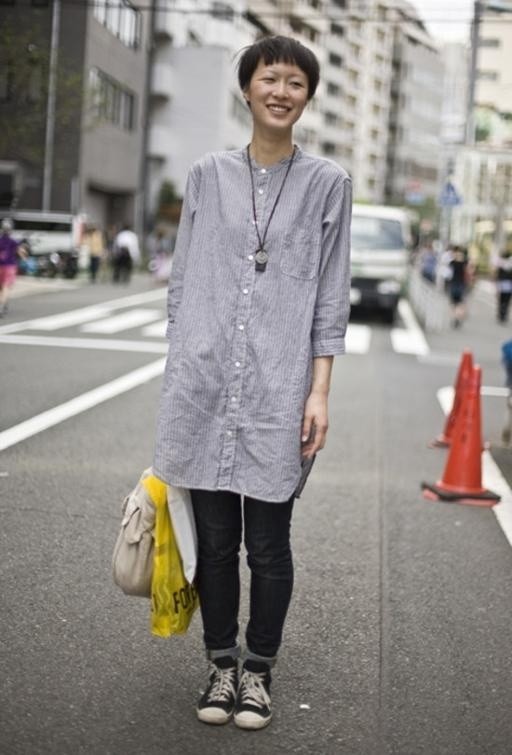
[[196, 656, 272, 730]]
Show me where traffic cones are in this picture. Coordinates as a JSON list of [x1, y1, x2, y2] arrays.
[[433, 352, 487, 447], [423, 371, 502, 505]]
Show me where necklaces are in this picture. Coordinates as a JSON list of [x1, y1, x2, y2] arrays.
[[246, 141, 299, 272]]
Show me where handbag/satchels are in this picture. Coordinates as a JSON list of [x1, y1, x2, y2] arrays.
[[112, 466, 200, 638]]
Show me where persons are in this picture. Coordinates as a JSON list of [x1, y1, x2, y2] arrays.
[[148, 36, 356, 732], [409, 215, 511, 331], [0, 216, 175, 319]]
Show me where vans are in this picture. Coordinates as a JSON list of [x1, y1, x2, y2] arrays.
[[344, 203, 411, 323]]
